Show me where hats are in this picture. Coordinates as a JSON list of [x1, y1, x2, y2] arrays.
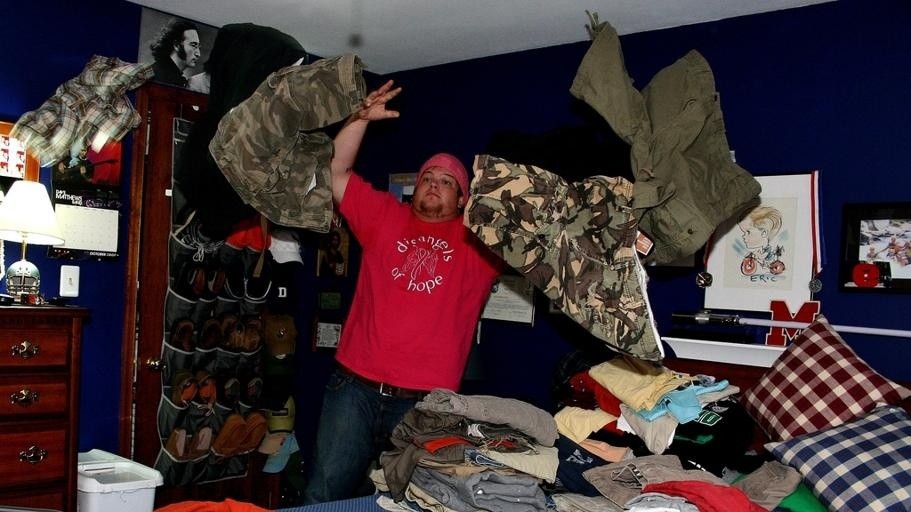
[[267, 392, 297, 434], [259, 431, 301, 475], [270, 228, 306, 268], [415, 152, 470, 208], [266, 266, 300, 314], [266, 314, 300, 357]]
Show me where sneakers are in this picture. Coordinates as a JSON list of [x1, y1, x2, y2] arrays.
[[221, 311, 245, 353], [224, 213, 266, 253], [167, 317, 195, 353], [173, 210, 227, 251], [240, 376, 266, 406], [196, 318, 222, 350], [245, 315, 263, 355], [219, 376, 242, 409], [212, 413, 268, 457]]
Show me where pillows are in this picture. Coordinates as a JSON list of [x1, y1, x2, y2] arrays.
[[762, 406, 911, 512], [737, 315, 911, 443]]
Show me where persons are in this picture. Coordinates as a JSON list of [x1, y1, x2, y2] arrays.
[[138, 14, 204, 91], [297, 77, 510, 506]]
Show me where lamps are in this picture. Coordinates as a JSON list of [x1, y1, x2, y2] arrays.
[[0, 180, 65, 304]]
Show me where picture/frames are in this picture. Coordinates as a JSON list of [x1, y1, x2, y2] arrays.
[[840, 201, 911, 296]]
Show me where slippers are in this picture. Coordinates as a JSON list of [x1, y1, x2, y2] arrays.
[[246, 260, 274, 302], [219, 257, 246, 302]]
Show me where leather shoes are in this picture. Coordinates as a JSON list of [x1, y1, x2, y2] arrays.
[[167, 368, 217, 406], [190, 424, 214, 460], [164, 426, 187, 461], [170, 254, 227, 302]]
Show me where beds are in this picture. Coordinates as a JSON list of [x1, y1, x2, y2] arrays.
[[269, 333, 911, 512]]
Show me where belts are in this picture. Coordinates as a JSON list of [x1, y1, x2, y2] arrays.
[[333, 360, 431, 403]]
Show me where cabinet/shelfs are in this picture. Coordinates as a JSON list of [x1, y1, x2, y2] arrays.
[[0, 303, 86, 512]]
